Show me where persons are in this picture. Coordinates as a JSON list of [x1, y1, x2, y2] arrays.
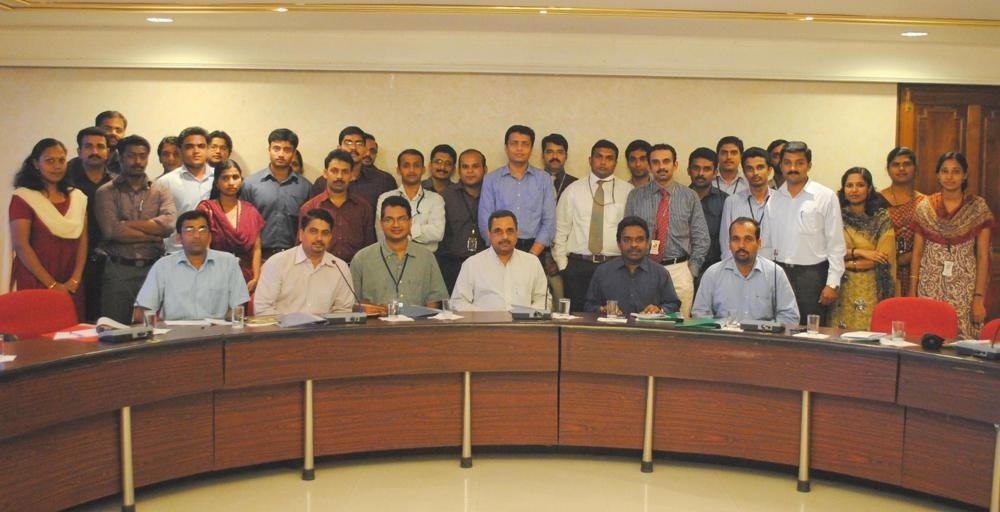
[[7, 109, 996, 340]]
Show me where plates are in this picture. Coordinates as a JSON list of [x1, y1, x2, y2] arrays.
[[632, 312, 667, 321]]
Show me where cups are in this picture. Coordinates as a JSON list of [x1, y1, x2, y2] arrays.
[[891, 319, 905, 340], [143, 310, 157, 330], [806, 313, 822, 335], [387, 302, 399, 317], [607, 300, 618, 318], [230, 305, 245, 329], [558, 297, 571, 315], [442, 297, 454, 315]]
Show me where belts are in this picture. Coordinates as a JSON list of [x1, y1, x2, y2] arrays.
[[569, 253, 619, 264], [659, 256, 687, 266], [111, 258, 157, 268]]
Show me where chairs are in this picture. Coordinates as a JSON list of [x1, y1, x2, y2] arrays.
[[872, 298, 957, 345], [1, 290, 80, 339], [979, 320, 1000, 342], [248, 294, 254, 316]]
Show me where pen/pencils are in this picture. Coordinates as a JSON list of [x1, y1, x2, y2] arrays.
[[847, 338, 880, 343], [201, 322, 215, 330]]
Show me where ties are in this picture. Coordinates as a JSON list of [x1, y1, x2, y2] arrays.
[[655, 189, 669, 262], [589, 180, 604, 255]]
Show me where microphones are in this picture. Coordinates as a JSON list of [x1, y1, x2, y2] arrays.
[[740, 248, 786, 332], [326, 259, 368, 325], [93, 246, 154, 343], [952, 323, 1000, 360], [511, 256, 554, 319]]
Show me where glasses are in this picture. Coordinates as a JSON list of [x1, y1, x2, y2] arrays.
[[181, 227, 209, 234], [384, 216, 409, 224]]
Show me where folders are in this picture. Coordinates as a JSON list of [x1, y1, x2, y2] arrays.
[[507, 308, 552, 321], [272, 309, 330, 329], [672, 318, 726, 330], [396, 304, 444, 320], [321, 311, 367, 326]]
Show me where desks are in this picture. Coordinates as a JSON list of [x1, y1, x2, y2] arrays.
[[898, 346, 1000, 511], [1, 322, 224, 511], [215, 309, 560, 482], [558, 316, 904, 493]]
